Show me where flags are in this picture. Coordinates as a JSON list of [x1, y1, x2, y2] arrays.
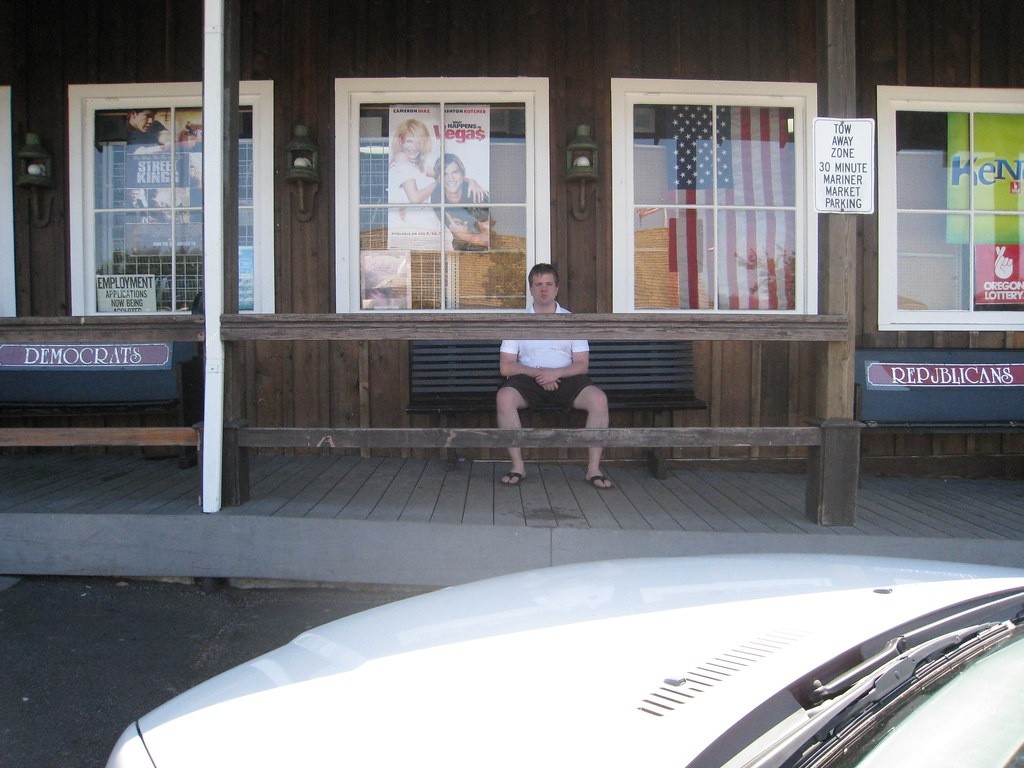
[[665, 106, 792, 311]]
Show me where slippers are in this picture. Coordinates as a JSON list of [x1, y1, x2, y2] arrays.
[[504, 472, 527, 487], [586, 475, 614, 489]]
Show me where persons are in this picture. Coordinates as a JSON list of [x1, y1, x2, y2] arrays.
[[495, 263, 613, 490], [125, 189, 145, 223], [115, 109, 172, 152], [388, 120, 489, 250]]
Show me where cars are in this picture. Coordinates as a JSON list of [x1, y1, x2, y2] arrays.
[[98, 551, 1018, 768]]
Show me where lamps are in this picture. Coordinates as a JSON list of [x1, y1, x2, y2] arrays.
[[17, 134, 55, 227], [565, 124, 602, 220], [284, 125, 321, 222]]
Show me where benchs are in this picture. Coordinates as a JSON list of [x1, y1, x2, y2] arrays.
[[406, 339, 708, 480], [0, 341, 206, 467], [844, 349, 1024, 489]]
